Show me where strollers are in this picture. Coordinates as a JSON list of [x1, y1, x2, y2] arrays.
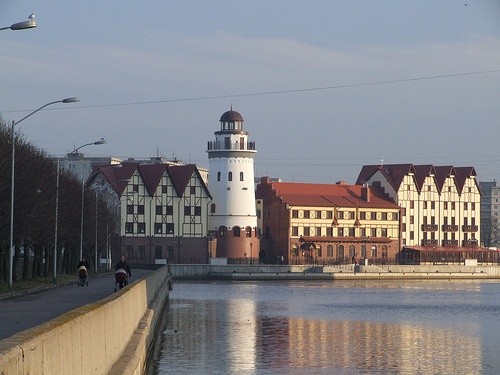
[[77, 266, 92, 286], [112, 269, 129, 293]]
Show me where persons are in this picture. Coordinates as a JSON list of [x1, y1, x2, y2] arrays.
[[114, 255, 131, 287], [78, 258, 90, 287]]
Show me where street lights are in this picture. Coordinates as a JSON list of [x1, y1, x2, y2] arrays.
[[94, 179, 130, 274], [107, 196, 136, 270], [79, 164, 121, 274], [0, 20, 36, 33], [7, 97, 81, 289], [54, 140, 106, 283]]
